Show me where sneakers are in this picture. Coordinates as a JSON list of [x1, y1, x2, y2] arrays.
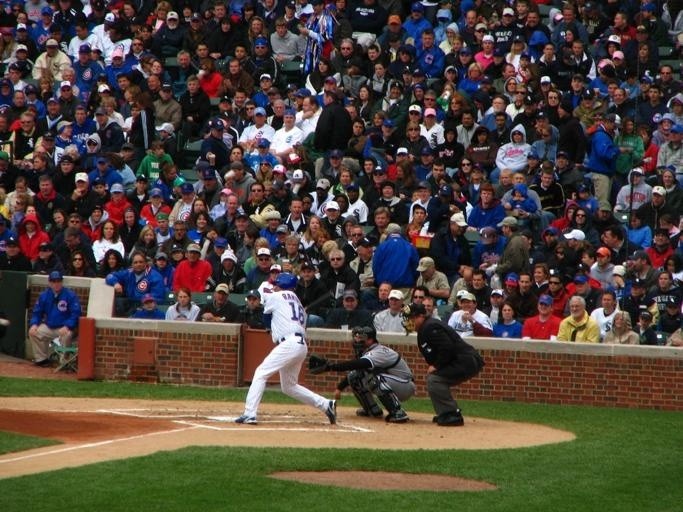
[[236, 416, 257, 424], [386, 411, 409, 422], [326, 400, 336, 424], [356, 408, 383, 419], [36, 361, 50, 367], [433, 416, 439, 423], [438, 413, 463, 426]]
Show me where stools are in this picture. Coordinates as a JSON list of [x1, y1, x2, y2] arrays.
[[52, 345, 78, 374]]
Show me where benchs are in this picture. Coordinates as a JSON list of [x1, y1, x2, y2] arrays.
[[160, 52, 305, 181]]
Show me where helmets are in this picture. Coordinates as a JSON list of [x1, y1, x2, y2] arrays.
[[352, 327, 377, 355], [400, 304, 426, 333]]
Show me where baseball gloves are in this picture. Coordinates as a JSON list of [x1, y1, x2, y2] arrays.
[[307, 355, 331, 374]]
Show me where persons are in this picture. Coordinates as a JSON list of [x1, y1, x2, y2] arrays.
[[234, 268, 339, 425], [400, 303, 490, 427], [307, 325, 415, 423], [21, 264, 88, 380], [0, 0, 682, 345]]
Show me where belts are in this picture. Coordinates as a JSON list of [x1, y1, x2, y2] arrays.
[[276, 333, 302, 344]]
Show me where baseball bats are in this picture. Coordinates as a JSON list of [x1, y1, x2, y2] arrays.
[[265, 290, 334, 334]]
